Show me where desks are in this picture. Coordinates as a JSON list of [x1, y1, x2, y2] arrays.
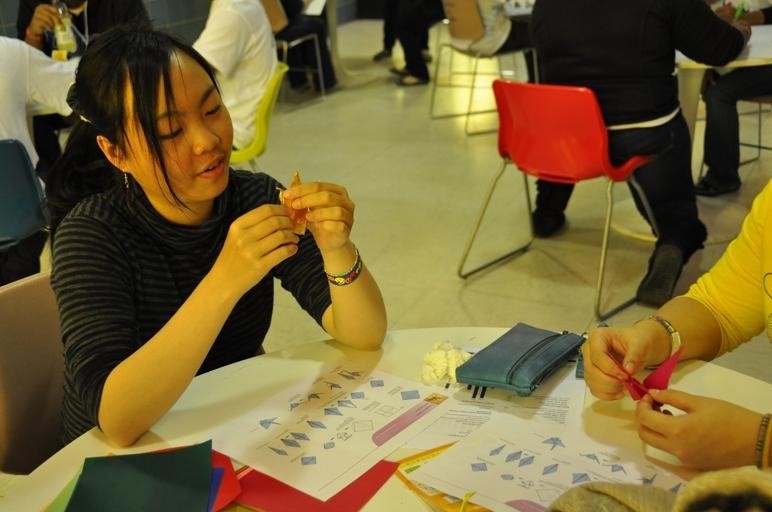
[[27, 324, 772, 512]]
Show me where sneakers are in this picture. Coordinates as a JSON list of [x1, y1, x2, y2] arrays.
[[294, 50, 435, 93], [634, 241, 689, 311], [531, 205, 570, 236], [690, 167, 741, 194]]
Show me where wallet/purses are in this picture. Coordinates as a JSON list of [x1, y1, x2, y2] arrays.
[[455, 318, 586, 398]]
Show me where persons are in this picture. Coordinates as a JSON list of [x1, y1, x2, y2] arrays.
[[695, 0, 772, 197], [579, 180, 772, 471], [34, 25, 387, 450], [17, 0, 145, 157], [528, 0, 752, 308], [191, 0, 278, 151], [0, 34, 81, 285], [262, 0, 535, 94]]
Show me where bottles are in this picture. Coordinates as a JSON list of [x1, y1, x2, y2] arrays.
[[50, 1, 78, 54]]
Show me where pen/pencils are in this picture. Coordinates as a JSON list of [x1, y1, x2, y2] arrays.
[[732, 1, 744, 23]]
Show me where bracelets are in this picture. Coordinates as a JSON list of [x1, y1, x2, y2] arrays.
[[762, 417, 772, 468], [755, 414, 770, 468], [324, 248, 363, 286], [633, 314, 681, 369], [26, 29, 41, 42]]
